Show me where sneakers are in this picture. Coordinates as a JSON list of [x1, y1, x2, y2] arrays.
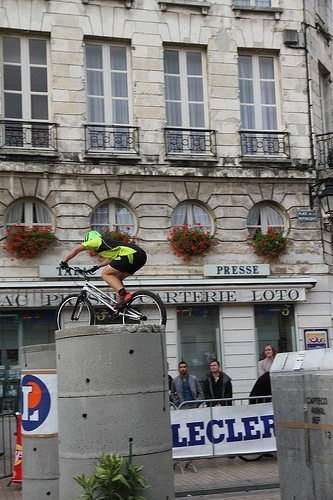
[[114, 293, 132, 309]]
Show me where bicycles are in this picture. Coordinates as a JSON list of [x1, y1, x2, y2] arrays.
[[57, 261, 167, 332]]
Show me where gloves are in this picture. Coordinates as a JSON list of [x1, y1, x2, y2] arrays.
[[60, 261, 68, 270], [89, 266, 99, 273]]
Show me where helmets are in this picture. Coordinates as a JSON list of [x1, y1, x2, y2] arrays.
[[84, 230, 102, 250]]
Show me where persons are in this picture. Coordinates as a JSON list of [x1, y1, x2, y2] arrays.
[[167, 361, 204, 409], [258, 344, 277, 376], [248, 371, 272, 404], [60, 231, 148, 309], [203, 360, 232, 407]]
